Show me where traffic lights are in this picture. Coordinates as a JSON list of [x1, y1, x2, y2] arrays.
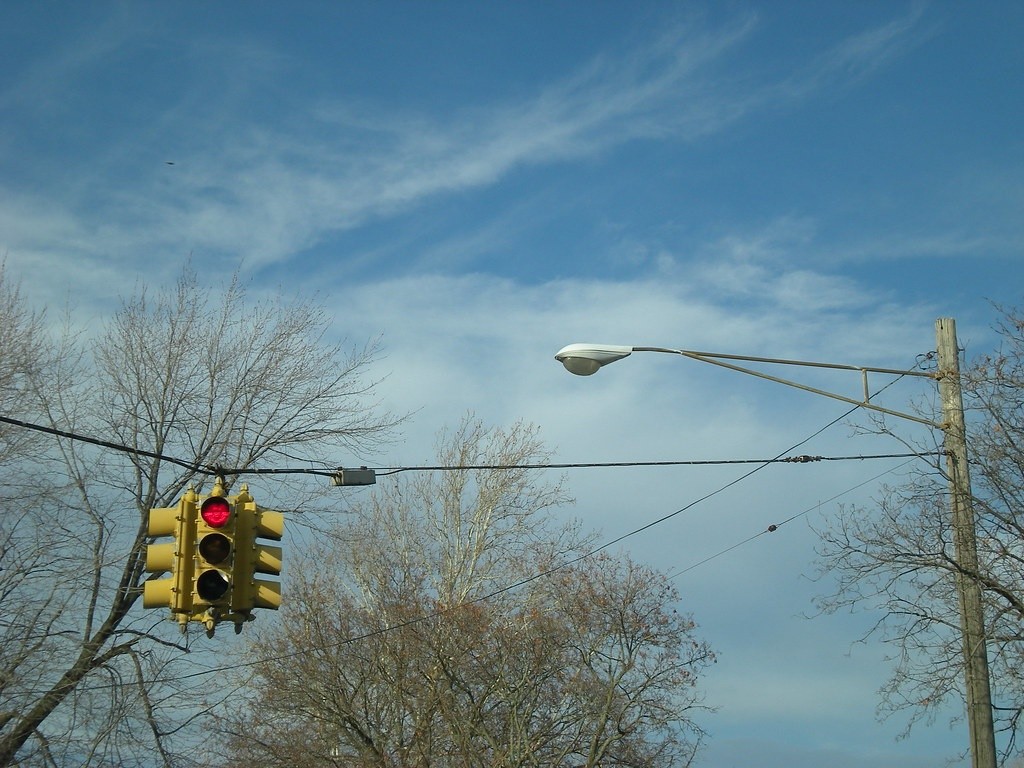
[[240, 484, 283, 631], [184, 478, 239, 638], [140, 492, 185, 626]]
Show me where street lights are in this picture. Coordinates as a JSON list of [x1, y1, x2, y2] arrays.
[[553, 316, 998, 768]]
